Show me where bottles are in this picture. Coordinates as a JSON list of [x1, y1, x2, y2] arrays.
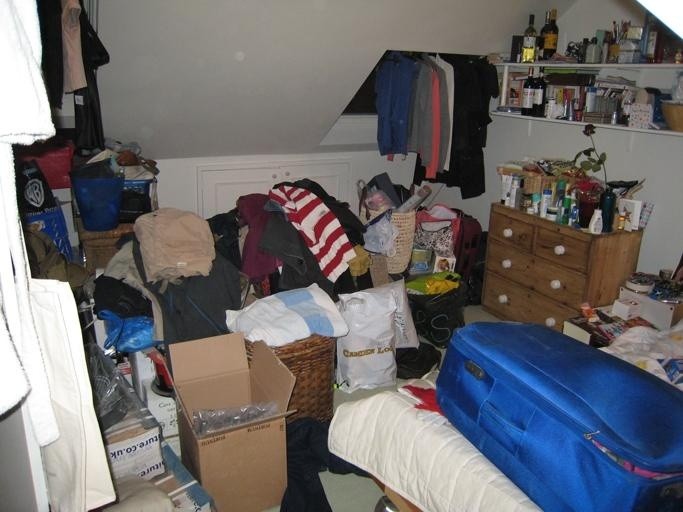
[[577, 30, 611, 63], [520, 7, 559, 64], [554, 181, 577, 227], [674, 70, 682, 100], [611, 206, 625, 232], [609, 110, 617, 125], [599, 187, 614, 233], [645, 23, 662, 64], [621, 99, 632, 126], [587, 207, 602, 235], [518, 65, 546, 117], [503, 174, 524, 211]]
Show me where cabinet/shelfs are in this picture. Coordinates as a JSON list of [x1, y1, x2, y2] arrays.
[[482, 203, 644, 333], [490, 62, 683, 136]]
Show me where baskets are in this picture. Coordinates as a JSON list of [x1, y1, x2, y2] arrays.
[[358, 183, 416, 275], [85, 237, 120, 247], [82, 238, 123, 274], [225, 329, 336, 425], [72, 215, 134, 241], [494, 166, 590, 209]]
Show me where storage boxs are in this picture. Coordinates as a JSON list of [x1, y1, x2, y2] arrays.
[[104, 332, 296, 511], [13, 128, 79, 262]]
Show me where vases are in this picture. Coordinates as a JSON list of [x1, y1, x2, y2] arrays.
[[599, 187, 618, 233]]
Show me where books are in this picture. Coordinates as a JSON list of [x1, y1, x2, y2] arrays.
[[541, 66, 639, 125]]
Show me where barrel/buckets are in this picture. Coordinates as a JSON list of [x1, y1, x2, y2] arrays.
[[70, 172, 126, 233]]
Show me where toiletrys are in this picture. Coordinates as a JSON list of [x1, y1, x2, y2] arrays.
[[500, 174, 526, 210], [532, 178, 580, 227], [588, 208, 604, 234], [585, 86, 597, 113]]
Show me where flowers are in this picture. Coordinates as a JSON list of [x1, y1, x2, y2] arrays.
[[572, 124, 609, 192]]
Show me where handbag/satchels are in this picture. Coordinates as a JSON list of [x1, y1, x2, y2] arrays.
[[334, 291, 398, 394]]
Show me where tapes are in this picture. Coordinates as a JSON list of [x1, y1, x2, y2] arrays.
[[626, 280, 655, 292]]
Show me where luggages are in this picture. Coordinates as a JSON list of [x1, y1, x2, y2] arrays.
[[436, 322, 683, 512], [450, 208, 482, 284]]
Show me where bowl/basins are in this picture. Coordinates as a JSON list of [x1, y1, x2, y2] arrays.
[[659, 102, 682, 133]]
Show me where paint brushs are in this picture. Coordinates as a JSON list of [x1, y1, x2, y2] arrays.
[[613, 20, 631, 44]]
